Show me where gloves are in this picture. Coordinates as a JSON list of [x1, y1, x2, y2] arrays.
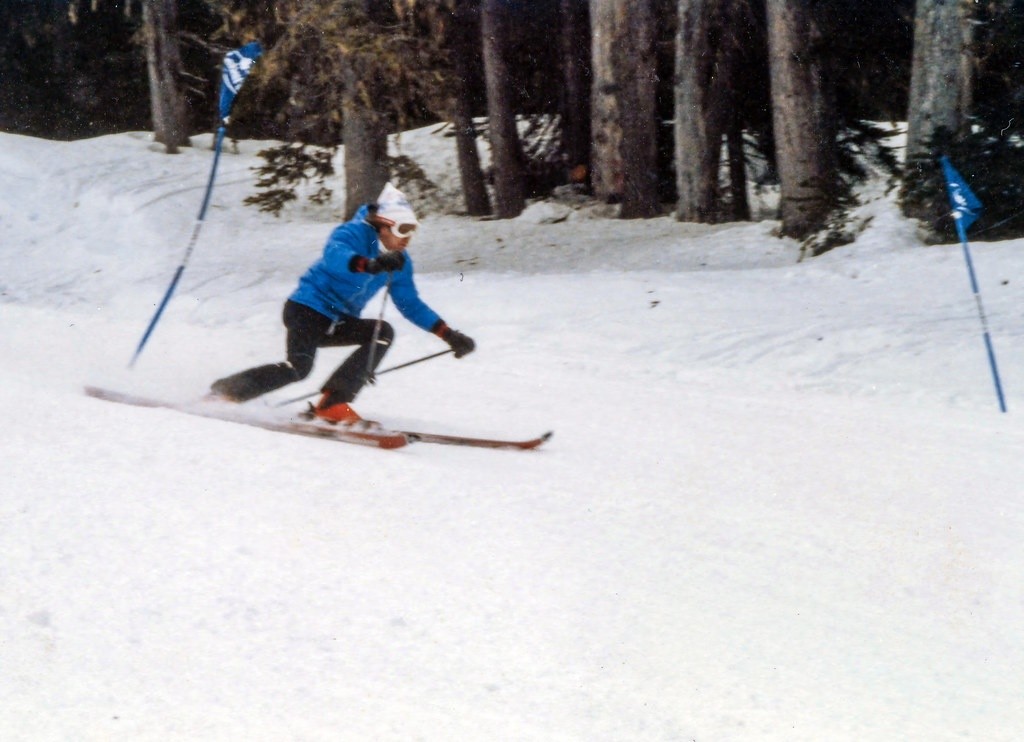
[[441, 328, 475, 358], [365, 251, 405, 273]]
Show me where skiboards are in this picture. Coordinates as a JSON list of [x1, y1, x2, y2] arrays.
[[84, 371, 553, 451]]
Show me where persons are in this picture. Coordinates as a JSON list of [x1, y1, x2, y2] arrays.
[[209, 181, 476, 431]]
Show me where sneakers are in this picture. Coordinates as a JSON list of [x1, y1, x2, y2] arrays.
[[318, 391, 363, 425]]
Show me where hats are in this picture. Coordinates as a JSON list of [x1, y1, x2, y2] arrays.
[[376, 182, 420, 226]]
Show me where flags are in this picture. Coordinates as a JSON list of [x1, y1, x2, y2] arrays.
[[939, 158, 984, 231], [218, 40, 263, 122]]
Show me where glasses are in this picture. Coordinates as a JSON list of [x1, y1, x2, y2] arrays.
[[391, 221, 417, 239]]
